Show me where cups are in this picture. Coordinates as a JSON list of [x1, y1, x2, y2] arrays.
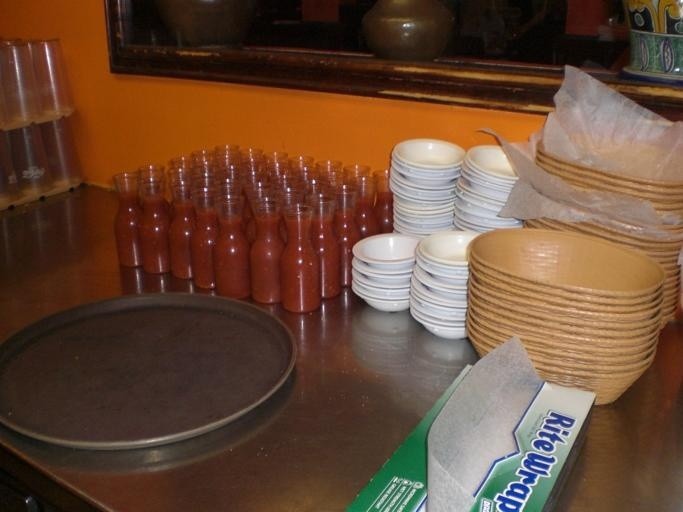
[[0, 35, 85, 211]]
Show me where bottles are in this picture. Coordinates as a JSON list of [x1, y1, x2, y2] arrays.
[[110, 143, 395, 317]]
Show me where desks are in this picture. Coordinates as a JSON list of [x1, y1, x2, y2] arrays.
[[0, 186, 683, 511]]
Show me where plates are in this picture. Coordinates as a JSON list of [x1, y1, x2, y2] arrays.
[[466, 127, 683, 407], [351, 136, 535, 340]]
